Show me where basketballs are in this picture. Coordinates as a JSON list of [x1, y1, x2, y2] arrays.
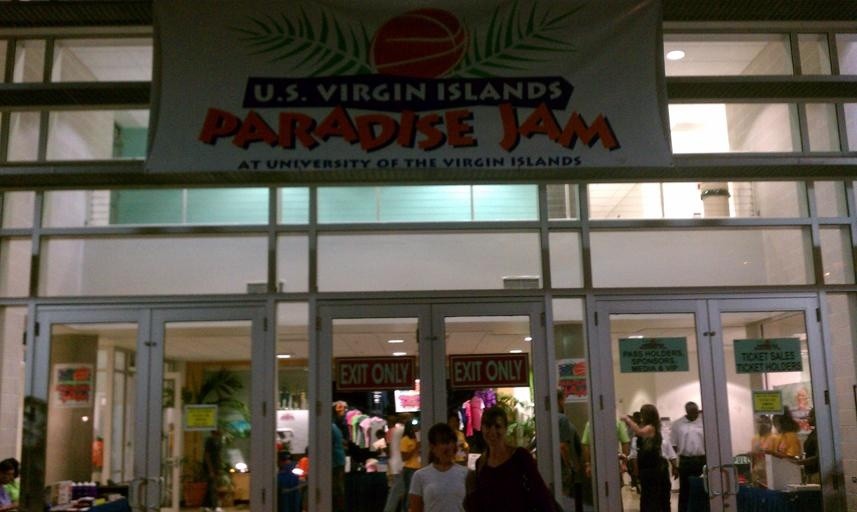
[[369, 8, 471, 78]]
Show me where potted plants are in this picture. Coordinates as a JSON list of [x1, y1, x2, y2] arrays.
[[161, 367, 252, 510]]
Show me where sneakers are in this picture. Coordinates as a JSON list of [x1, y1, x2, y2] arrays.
[[203, 506, 223, 512]]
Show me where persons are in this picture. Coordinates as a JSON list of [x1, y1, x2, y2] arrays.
[[622, 404, 679, 512], [582, 419, 630, 512], [276, 407, 381, 512], [476, 407, 563, 512], [751, 414, 784, 469], [198, 427, 224, 512], [759, 401, 802, 490], [0, 460, 25, 512], [558, 390, 585, 512], [791, 408, 821, 484], [388, 404, 420, 512], [409, 422, 476, 512], [671, 402, 709, 512], [447, 412, 471, 466], [2, 457, 20, 511]]
